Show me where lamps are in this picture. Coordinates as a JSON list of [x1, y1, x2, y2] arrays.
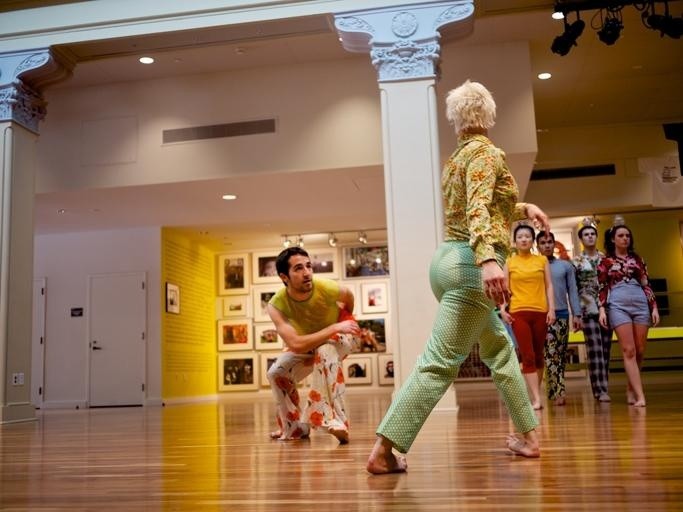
[[545, 2, 683, 57], [279, 226, 388, 250]]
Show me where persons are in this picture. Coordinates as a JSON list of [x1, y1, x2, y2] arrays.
[[594, 224, 660, 408], [602, 212, 632, 258], [266, 247, 360, 445], [223, 249, 394, 384], [368, 407, 667, 511], [536, 231, 581, 407], [498, 224, 554, 409], [569, 225, 613, 404], [364, 76, 550, 475]]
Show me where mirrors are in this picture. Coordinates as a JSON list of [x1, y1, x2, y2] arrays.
[[528, 208, 683, 332]]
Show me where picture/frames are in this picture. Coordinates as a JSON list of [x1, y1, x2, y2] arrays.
[[165, 282, 180, 315], [215, 242, 394, 392]]
